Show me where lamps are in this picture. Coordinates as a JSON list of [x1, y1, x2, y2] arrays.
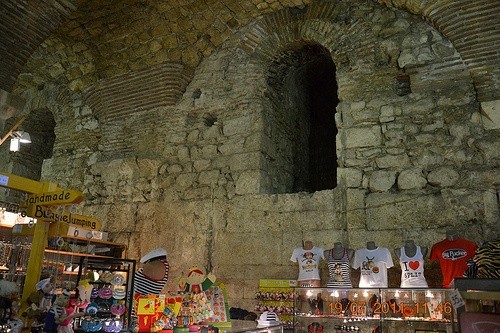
[[8, 129, 32, 153]]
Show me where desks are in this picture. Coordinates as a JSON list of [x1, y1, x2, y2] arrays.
[[159, 318, 282, 333]]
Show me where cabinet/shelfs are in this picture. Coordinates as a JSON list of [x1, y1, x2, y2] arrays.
[[255, 278, 296, 333], [1, 233, 127, 297], [378, 288, 456, 333], [294, 288, 382, 333], [76, 257, 136, 333]]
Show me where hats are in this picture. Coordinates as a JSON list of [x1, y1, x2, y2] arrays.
[[140, 249, 167, 264]]
